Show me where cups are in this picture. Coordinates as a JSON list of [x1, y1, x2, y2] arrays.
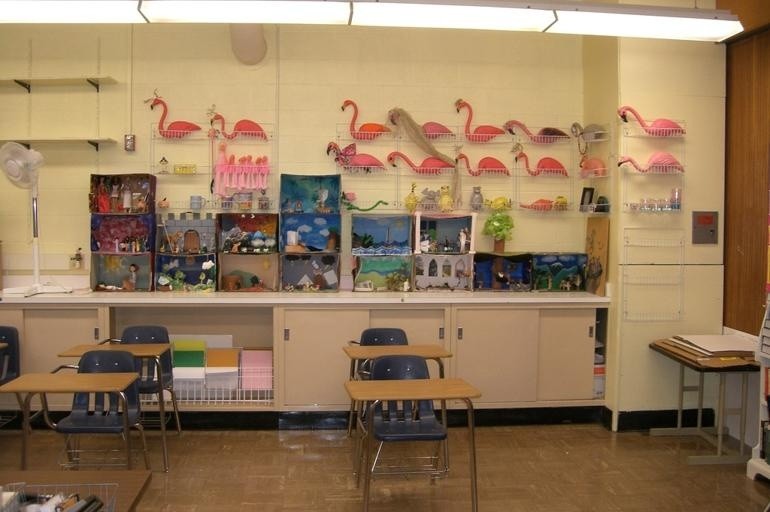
[[624, 186, 683, 214], [287, 230, 299, 246], [190, 195, 206, 210], [219, 193, 253, 209]]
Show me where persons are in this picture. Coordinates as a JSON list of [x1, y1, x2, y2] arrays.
[[96, 177, 134, 213], [114, 236, 142, 252], [127, 263, 138, 290]]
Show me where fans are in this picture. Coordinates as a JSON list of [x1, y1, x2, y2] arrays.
[[0, 140, 73, 296]]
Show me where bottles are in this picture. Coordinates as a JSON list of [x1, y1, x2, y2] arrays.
[[471, 186, 484, 211]]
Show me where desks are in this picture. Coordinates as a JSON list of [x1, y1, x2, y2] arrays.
[[647, 343, 762, 466], [338, 378, 484, 511]]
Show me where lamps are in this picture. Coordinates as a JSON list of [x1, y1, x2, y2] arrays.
[[140, 2, 745, 45]]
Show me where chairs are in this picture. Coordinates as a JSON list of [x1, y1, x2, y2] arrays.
[[346, 327, 453, 486], [0, 326, 184, 471]]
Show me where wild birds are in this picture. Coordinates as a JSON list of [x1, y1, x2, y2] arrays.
[[323, 96, 687, 177], [148, 99, 201, 139], [208, 114, 268, 143]]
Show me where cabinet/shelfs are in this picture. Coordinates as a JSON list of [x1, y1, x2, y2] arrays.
[[149, 122, 280, 214], [335, 122, 614, 217], [624, 120, 686, 324], [0, 72, 120, 152], [0, 305, 100, 413], [282, 305, 451, 405], [451, 303, 607, 406]]
